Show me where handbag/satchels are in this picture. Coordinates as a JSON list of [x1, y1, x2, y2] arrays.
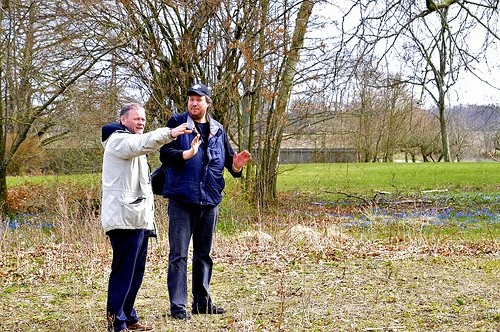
[[150, 163, 166, 195]]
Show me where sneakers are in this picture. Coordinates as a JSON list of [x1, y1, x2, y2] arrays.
[[129, 322, 153, 330], [118, 328, 133, 332], [192, 305, 226, 315], [173, 311, 191, 320]]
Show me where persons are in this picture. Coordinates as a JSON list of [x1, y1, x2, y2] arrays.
[[160, 84, 252, 321], [101, 103, 192, 332]]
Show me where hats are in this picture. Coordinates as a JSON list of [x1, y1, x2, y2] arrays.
[[186, 84, 210, 96]]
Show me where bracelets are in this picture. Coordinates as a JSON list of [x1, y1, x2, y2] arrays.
[[232, 164, 242, 171]]
